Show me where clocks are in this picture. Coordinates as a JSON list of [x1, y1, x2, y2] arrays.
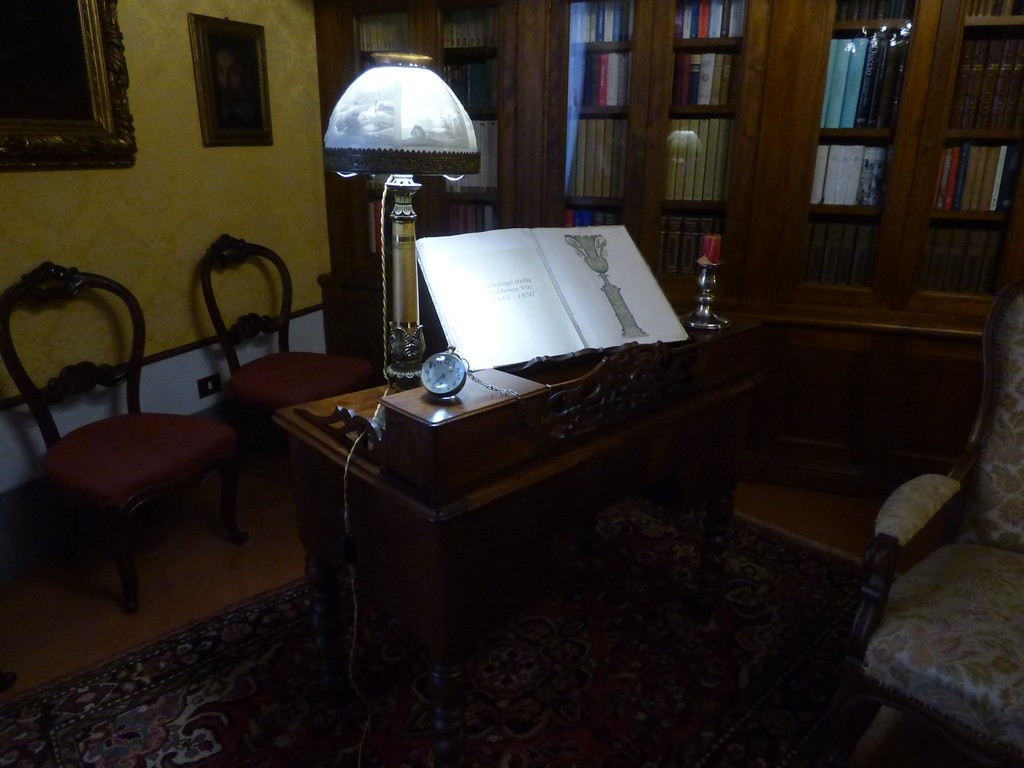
[[420, 347, 521, 405]]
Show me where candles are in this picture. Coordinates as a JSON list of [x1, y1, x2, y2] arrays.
[[702, 234, 721, 261]]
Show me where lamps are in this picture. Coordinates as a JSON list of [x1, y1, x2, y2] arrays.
[[326, 52, 483, 387]]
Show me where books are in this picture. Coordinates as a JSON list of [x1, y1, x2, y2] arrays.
[[442, 5, 497, 236], [806, 0, 918, 286], [368, 200, 392, 254], [359, 11, 407, 72], [921, 0, 1024, 297], [566, 0, 635, 228], [656, 0, 746, 272], [416, 226, 690, 374]]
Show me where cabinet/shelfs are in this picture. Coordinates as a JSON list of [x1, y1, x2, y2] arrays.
[[314, 0, 1024, 491]]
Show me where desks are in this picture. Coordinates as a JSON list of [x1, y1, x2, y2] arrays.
[[273, 363, 769, 767]]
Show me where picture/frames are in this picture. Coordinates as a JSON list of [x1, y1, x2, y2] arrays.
[[0, 0, 138, 171], [188, 12, 274, 148]]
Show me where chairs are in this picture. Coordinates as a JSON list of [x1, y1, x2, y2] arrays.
[[827, 275, 1024, 768], [1, 262, 248, 617], [197, 232, 374, 467]]
